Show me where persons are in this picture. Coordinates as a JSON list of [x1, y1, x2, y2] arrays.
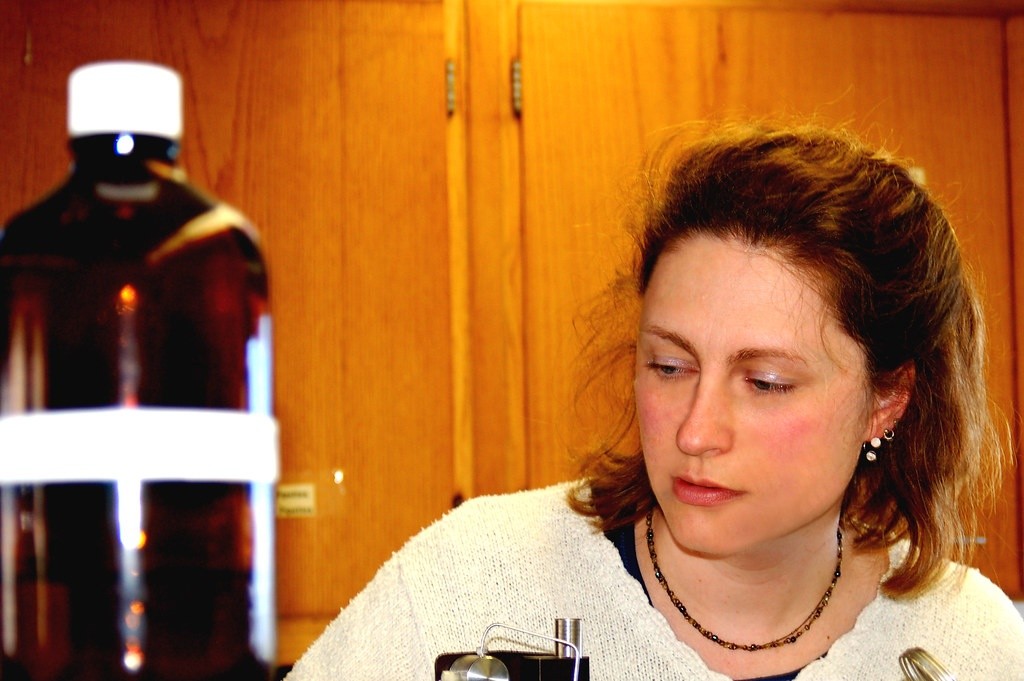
[[280, 126, 1024, 681]]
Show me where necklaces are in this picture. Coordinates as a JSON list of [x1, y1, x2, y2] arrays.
[[644, 501, 844, 652]]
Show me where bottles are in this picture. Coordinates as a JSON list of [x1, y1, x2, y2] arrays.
[[0, 61, 278, 681]]
